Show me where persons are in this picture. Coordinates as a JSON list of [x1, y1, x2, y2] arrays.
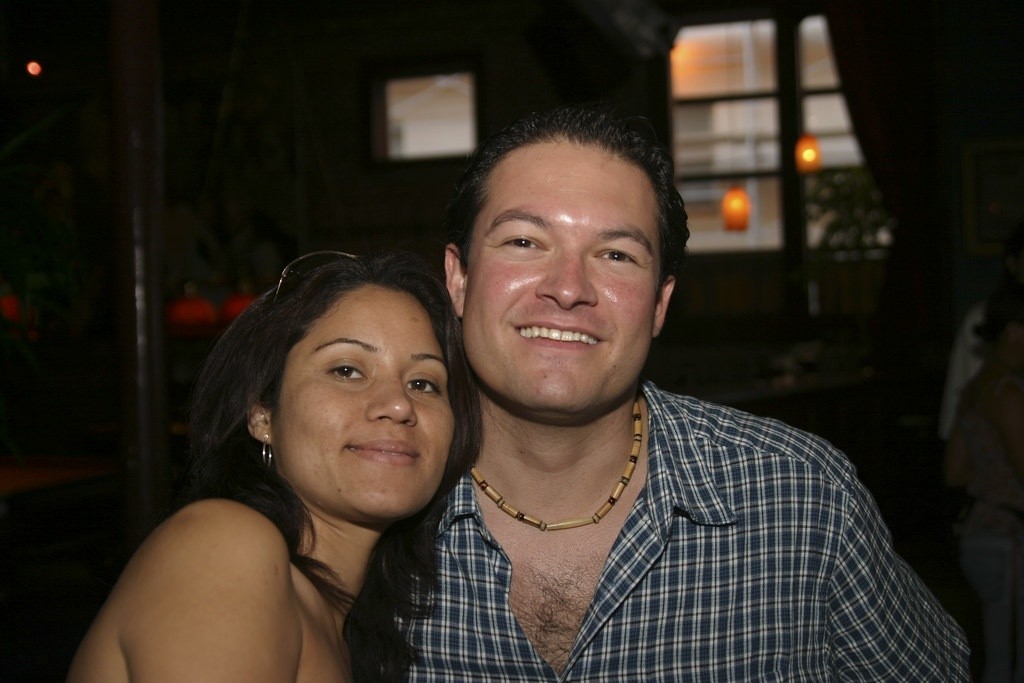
[[393, 113, 968, 683], [70, 251, 484, 683], [944, 316, 1024, 683]]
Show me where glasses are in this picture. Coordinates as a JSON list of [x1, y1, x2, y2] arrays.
[[273, 250, 362, 303]]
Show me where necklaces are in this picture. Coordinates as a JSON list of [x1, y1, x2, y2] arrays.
[[471, 402, 642, 532]]
